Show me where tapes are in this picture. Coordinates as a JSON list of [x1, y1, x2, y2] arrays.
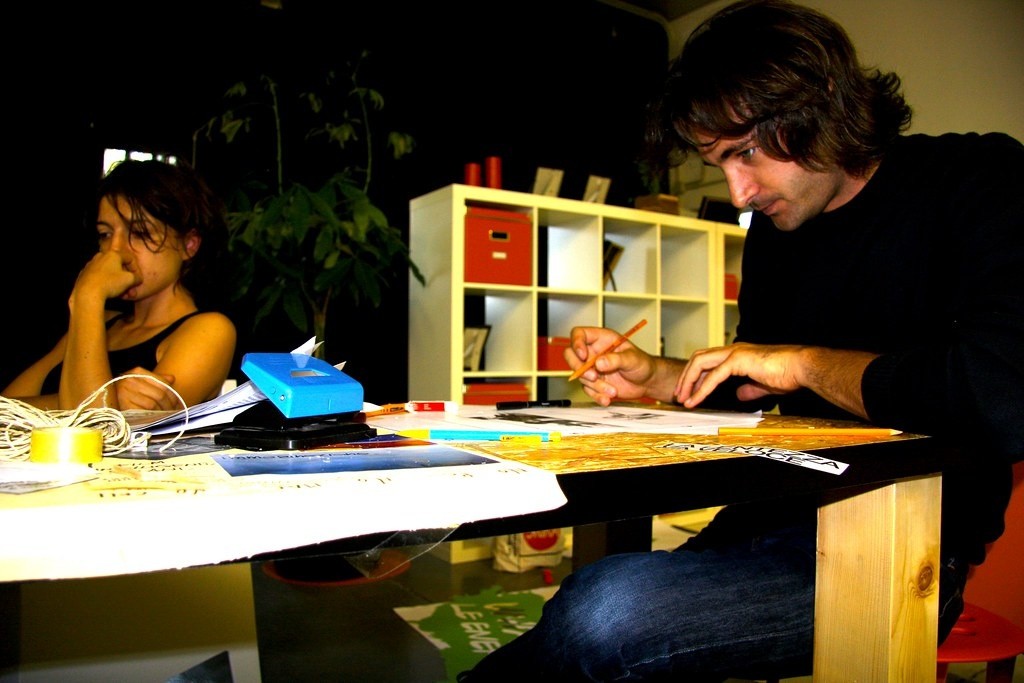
[[29, 427, 104, 463]]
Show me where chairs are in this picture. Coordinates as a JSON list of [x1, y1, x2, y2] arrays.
[[937, 458, 1024, 683]]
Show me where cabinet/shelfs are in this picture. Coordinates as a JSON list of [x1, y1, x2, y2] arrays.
[[408, 183, 749, 403]]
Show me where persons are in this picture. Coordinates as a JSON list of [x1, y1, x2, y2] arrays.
[[455, 0, 1024, 683], [1, 159, 236, 411]]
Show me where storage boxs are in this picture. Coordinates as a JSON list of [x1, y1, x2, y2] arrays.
[[538, 336, 576, 371], [464, 207, 534, 285]]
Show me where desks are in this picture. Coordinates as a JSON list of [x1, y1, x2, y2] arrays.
[[0, 410, 944, 683]]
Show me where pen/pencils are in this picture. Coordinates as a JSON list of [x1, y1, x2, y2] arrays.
[[496, 398, 571, 410], [569, 317, 647, 384], [717, 426, 902, 437]]
